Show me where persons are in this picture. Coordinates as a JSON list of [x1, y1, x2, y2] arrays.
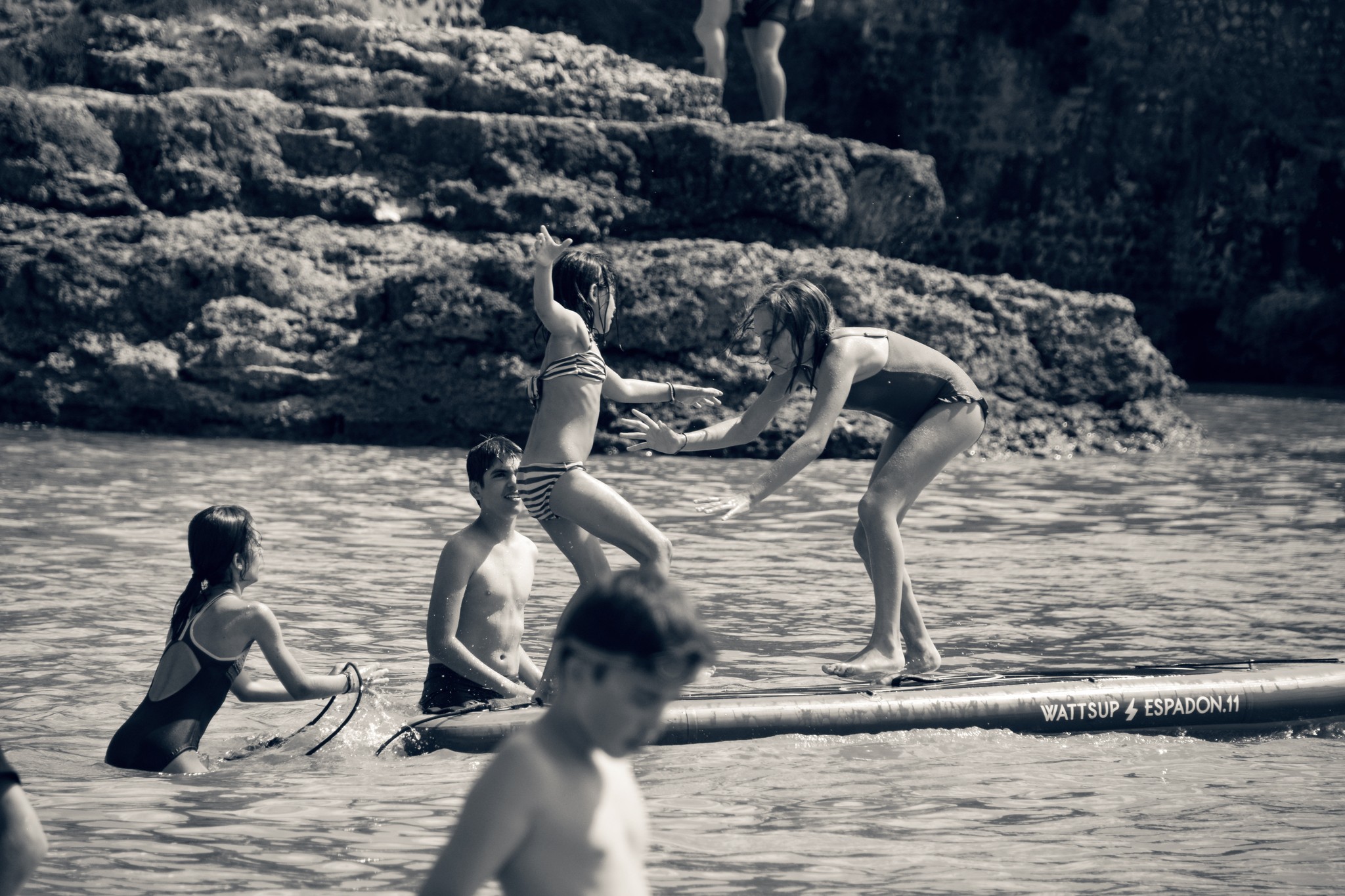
[[105, 505, 389, 774], [620, 278, 989, 685], [419, 437, 543, 715], [692, 0, 797, 128], [515, 225, 723, 704], [0, 748, 49, 896], [417, 566, 715, 896]]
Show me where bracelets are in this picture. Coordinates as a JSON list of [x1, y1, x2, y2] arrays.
[[666, 381, 676, 404], [673, 432, 688, 455], [342, 672, 355, 695]]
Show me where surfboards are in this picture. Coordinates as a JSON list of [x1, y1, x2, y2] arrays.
[[401, 656, 1344, 760]]
